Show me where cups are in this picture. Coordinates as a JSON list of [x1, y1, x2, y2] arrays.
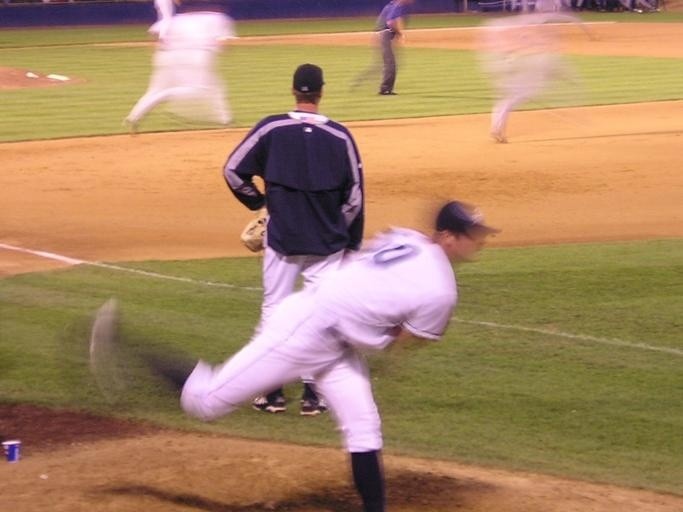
[[2, 440, 21, 464]]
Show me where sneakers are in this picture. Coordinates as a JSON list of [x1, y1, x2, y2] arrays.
[[299, 397, 330, 417], [251, 392, 287, 414]]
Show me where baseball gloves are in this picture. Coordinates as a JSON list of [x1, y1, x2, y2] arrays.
[[241, 218, 265, 252]]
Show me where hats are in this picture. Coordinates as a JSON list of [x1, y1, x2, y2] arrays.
[[293, 63, 325, 93], [435, 201, 503, 236]]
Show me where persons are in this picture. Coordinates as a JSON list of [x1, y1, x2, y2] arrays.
[[219, 63, 366, 418], [84, 199, 504, 512], [372, 1, 413, 97], [153, 1, 180, 24], [475, 0, 599, 149], [121, 1, 233, 143], [456, 0, 661, 15]]
